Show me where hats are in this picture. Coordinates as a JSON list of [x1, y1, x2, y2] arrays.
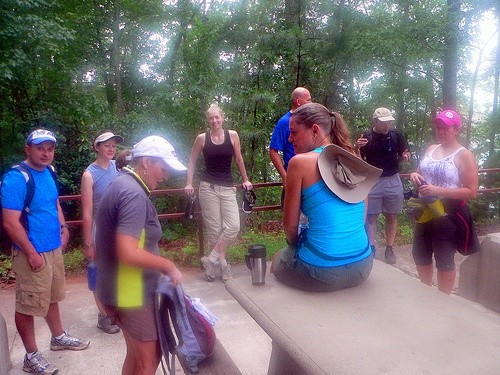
[[372, 107, 395, 122], [317, 144, 383, 203], [93, 132, 123, 149], [431, 110, 462, 127], [126, 135, 188, 171], [27, 129, 57, 147], [242, 189, 257, 214]]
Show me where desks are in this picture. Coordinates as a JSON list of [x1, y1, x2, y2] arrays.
[[224, 256, 500, 375]]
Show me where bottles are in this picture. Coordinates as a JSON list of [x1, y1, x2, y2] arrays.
[[421, 183, 445, 217], [186, 194, 197, 221], [87, 260, 97, 291]]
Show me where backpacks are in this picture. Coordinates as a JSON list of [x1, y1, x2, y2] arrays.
[[154, 273, 216, 373]]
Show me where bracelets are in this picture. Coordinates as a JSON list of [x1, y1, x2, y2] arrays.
[[59, 224, 69, 227]]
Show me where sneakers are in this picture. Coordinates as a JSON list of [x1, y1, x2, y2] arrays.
[[50, 329, 90, 351], [23, 351, 59, 375]]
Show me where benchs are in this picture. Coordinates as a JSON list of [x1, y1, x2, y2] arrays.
[[176, 336, 242, 375]]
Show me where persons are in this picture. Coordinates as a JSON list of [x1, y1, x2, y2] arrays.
[[185, 105, 253, 279], [1, 127, 90, 375], [272, 88, 311, 241], [355, 108, 410, 265], [91, 136, 184, 375], [270, 103, 373, 291], [80, 132, 125, 330], [406, 109, 479, 293]]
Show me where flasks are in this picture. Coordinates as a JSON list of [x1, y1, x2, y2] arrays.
[[244, 244, 267, 286]]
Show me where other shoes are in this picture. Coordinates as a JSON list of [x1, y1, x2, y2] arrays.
[[385, 248, 397, 264], [221, 264, 233, 282], [97, 313, 120, 333], [371, 246, 376, 257], [201, 257, 217, 281]]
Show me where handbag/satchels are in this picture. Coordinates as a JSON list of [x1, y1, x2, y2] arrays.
[[405, 197, 432, 223]]
